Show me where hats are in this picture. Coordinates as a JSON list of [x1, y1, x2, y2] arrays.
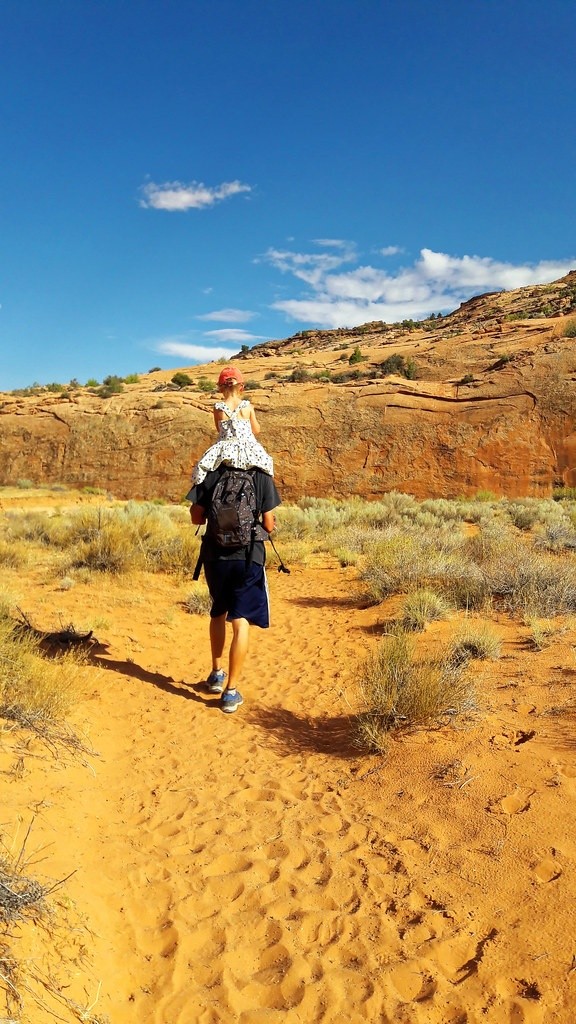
[[218, 367, 243, 387]]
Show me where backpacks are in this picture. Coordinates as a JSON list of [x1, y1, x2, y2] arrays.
[[211, 464, 269, 548]]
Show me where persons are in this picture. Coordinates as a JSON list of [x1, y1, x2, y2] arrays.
[[193, 367, 274, 483], [186, 465, 282, 713]]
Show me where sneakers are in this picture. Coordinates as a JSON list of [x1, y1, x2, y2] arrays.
[[206, 670, 227, 693], [221, 690, 243, 712]]
